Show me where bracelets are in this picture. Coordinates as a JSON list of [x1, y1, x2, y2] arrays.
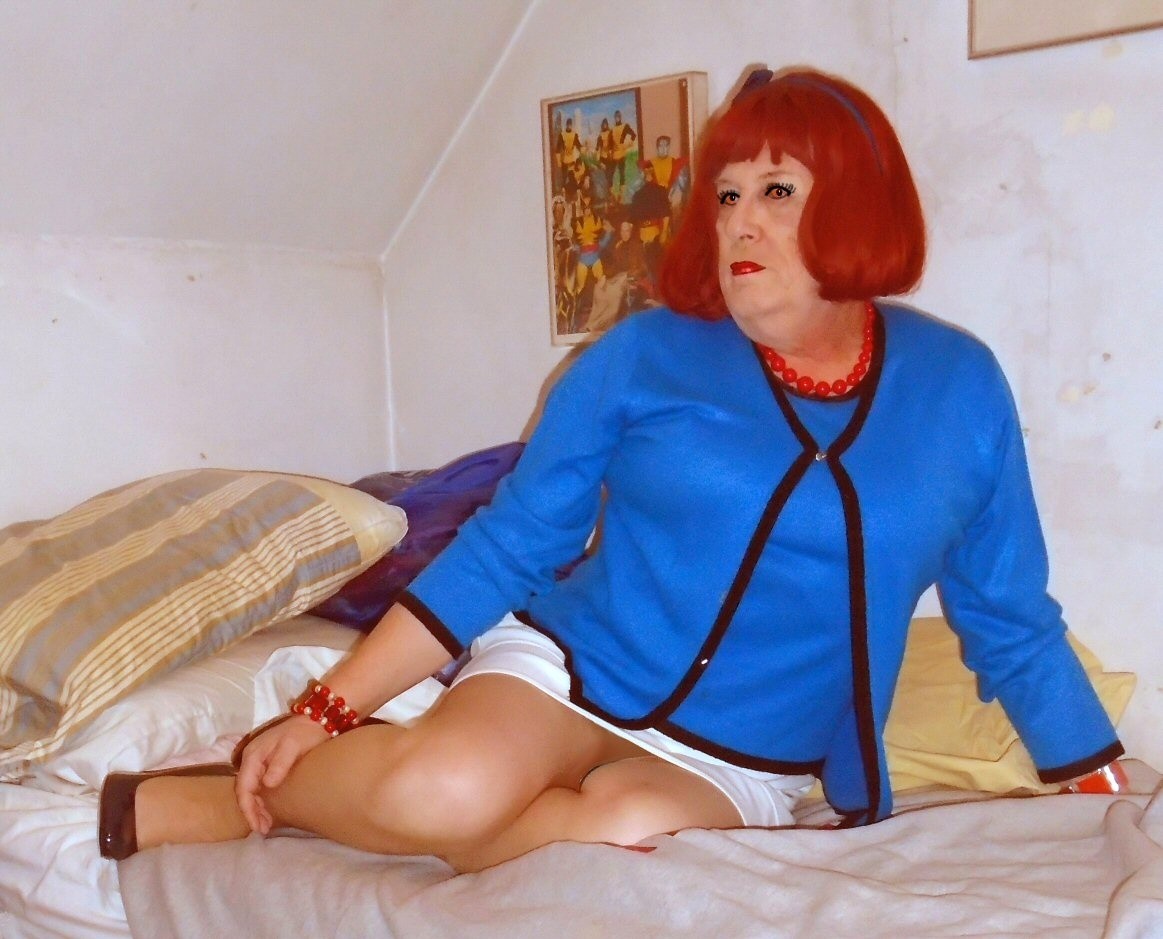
[[289, 678, 361, 740], [1060, 757, 1129, 797]]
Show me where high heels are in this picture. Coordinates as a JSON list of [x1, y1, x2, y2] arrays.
[[230, 714, 387, 774], [95, 763, 234, 861]]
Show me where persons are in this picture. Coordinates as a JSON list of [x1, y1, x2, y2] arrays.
[[101, 70, 1162, 873]]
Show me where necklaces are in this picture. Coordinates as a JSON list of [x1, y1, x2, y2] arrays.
[[756, 302, 876, 399]]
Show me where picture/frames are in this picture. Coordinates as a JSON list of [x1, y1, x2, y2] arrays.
[[967, 0, 1163, 61]]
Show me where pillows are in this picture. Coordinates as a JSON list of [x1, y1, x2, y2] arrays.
[[31, 609, 366, 795], [800, 618, 1137, 807], [0, 468, 410, 785]]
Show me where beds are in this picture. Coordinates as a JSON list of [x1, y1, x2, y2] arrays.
[[0, 676, 1163, 939]]
